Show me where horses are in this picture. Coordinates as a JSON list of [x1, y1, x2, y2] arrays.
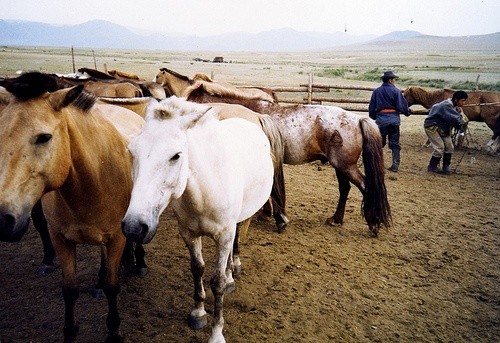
[[0, 67, 395, 342], [403, 86, 500, 146]]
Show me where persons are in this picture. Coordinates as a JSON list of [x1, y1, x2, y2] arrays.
[[369, 71, 411, 172], [424, 91, 468, 174]]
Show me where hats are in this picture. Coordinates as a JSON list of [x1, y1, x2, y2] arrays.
[[381, 71, 395, 78]]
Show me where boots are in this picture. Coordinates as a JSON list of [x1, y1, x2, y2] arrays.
[[443, 151, 452, 175], [428, 155, 442, 174], [387, 148, 400, 172]]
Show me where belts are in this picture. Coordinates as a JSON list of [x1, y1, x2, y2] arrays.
[[379, 108, 397, 113]]
